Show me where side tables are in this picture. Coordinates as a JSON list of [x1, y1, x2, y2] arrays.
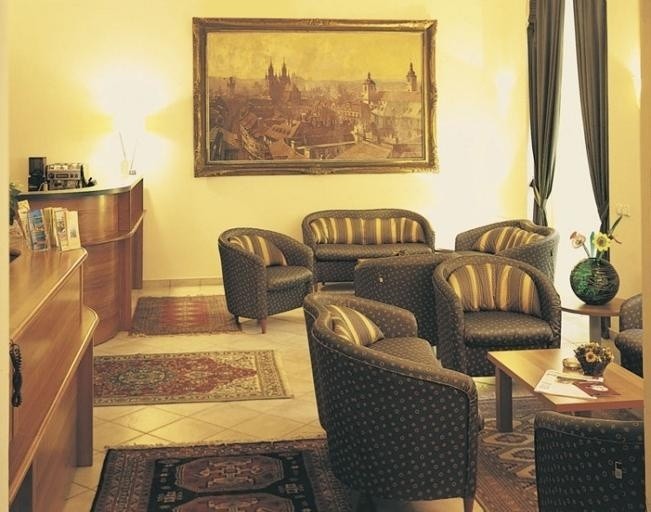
[[561, 296, 623, 339]]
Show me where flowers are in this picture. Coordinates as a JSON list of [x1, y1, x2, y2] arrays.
[[574, 341, 614, 363], [570, 205, 631, 287]]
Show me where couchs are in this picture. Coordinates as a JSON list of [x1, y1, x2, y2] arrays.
[[303, 294, 484, 511], [303, 209, 435, 283]]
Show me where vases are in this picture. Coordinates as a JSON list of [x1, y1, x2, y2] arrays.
[[570, 257, 619, 305], [575, 355, 610, 377]]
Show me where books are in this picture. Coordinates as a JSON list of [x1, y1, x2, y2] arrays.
[[534, 369, 604, 400], [572, 381, 621, 397]]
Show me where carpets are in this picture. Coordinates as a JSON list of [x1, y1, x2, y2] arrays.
[[89, 438, 354, 512], [92, 350, 291, 407], [130, 294, 241, 335], [476, 396, 644, 512]]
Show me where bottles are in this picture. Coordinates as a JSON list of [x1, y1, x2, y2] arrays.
[[129, 159, 136, 183]]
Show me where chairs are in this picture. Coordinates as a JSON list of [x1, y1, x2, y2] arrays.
[[615, 293, 643, 379], [435, 255, 562, 376], [219, 227, 315, 334], [534, 411, 646, 512], [354, 257, 440, 360], [455, 219, 559, 286]]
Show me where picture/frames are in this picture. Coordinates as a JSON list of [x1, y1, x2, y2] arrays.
[[190, 16, 436, 177]]
